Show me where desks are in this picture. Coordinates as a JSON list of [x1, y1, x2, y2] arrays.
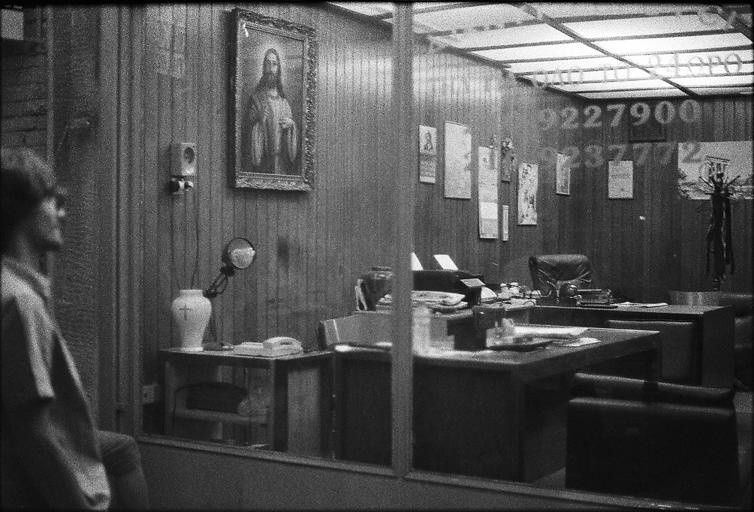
[[333, 319, 663, 480], [530, 297, 735, 390]]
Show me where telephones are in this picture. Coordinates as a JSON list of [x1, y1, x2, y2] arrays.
[[233, 336, 304, 357]]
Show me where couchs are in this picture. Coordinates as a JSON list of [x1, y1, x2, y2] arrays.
[[720, 291, 754, 390]]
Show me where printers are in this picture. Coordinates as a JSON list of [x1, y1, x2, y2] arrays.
[[412, 252, 485, 310]]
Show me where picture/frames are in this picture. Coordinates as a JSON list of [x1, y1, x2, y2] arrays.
[[229, 9, 320, 194]]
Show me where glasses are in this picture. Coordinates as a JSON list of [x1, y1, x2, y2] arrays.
[[46, 186, 68, 210]]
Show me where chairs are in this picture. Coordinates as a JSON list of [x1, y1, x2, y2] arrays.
[[529, 253, 594, 302], [666, 290, 720, 306], [317, 313, 392, 457], [565, 369, 754, 507]]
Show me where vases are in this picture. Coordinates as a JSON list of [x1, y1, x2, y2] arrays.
[[360, 266, 398, 312], [171, 289, 213, 353]]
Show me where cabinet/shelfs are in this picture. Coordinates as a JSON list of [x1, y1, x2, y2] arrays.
[[158, 344, 333, 447]]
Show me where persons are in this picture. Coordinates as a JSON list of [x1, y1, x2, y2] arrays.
[[241, 48, 297, 174], [424, 132, 432, 151], [0, 146, 150, 512]]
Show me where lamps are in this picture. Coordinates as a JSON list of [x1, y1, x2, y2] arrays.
[[201, 238, 256, 350]]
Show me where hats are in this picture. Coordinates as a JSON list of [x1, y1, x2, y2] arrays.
[[1, 147, 69, 219]]
